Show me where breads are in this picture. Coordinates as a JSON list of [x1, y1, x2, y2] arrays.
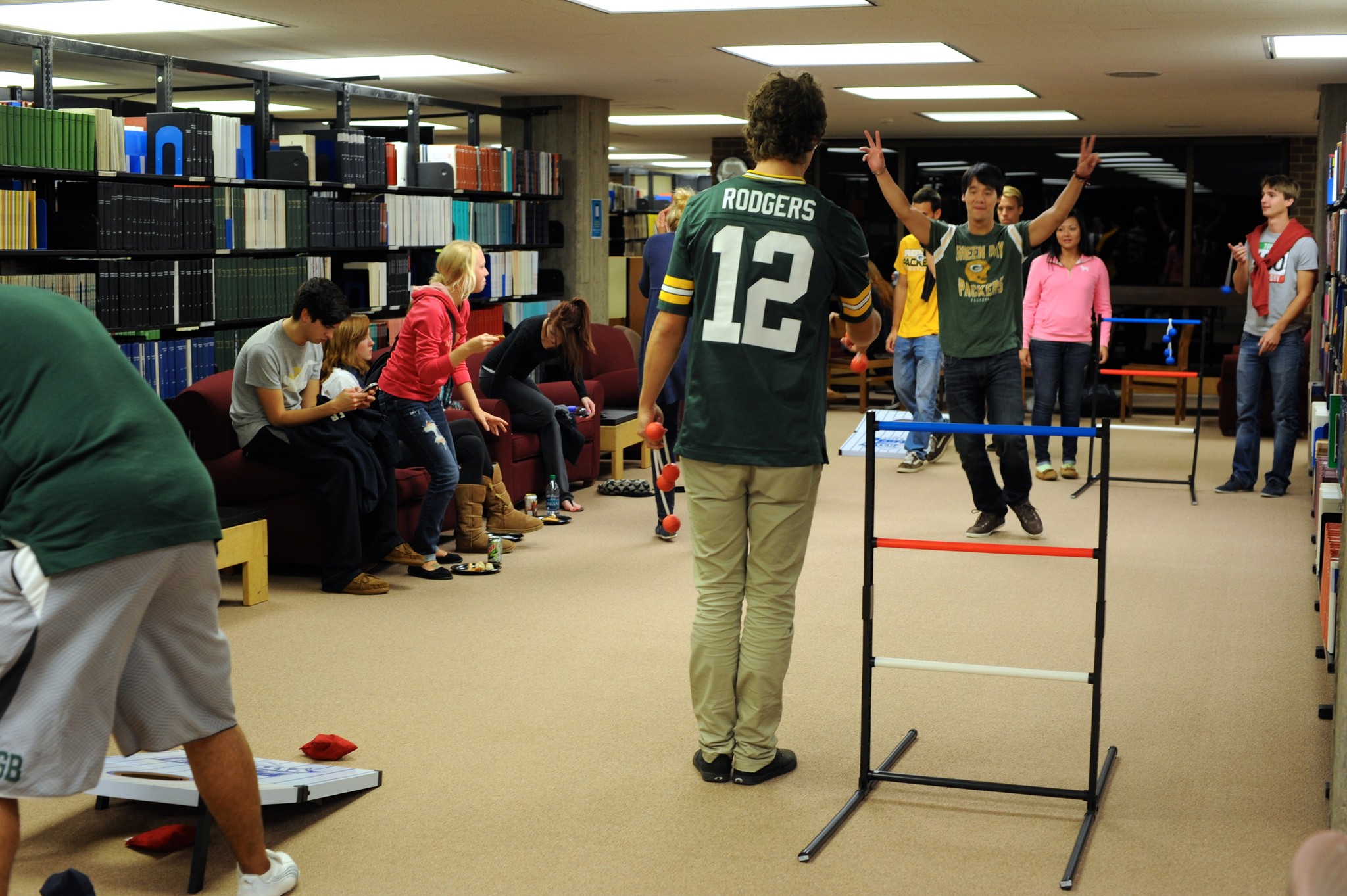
[[465, 561, 494, 571]]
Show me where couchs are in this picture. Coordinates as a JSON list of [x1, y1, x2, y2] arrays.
[[170, 322, 642, 544]]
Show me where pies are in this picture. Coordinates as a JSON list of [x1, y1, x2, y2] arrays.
[[542, 516, 559, 522]]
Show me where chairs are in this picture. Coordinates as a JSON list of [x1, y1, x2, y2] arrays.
[[1118, 321, 1196, 426]]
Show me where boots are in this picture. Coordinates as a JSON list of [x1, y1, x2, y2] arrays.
[[482, 461, 544, 534], [455, 483, 515, 553]]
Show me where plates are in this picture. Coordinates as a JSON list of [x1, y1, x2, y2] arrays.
[[533, 515, 572, 523], [450, 563, 502, 573], [483, 532, 525, 540]]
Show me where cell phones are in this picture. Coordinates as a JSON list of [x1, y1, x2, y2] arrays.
[[360, 382, 378, 394]]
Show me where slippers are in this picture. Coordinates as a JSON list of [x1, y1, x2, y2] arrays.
[[559, 499, 584, 512]]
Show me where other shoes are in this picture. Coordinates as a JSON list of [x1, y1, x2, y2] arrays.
[[1036, 469, 1058, 480], [407, 564, 453, 580], [1060, 464, 1079, 479], [987, 443, 998, 452], [436, 552, 463, 564]]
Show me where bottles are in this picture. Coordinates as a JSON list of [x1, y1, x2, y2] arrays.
[[565, 405, 591, 418], [545, 475, 560, 517]]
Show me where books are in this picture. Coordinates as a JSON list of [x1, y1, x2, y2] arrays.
[[0, 105, 239, 401], [1308, 131, 1347, 654], [237, 125, 457, 356], [608, 182, 658, 257], [418, 144, 563, 302]]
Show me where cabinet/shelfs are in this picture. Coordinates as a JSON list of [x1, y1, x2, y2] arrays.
[[1304, 104, 1347, 807], [0, 26, 572, 405], [608, 156, 713, 252]]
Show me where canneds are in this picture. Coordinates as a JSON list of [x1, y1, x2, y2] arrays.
[[523, 493, 537, 517], [487, 536, 502, 564]]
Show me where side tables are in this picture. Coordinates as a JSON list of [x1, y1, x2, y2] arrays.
[[599, 405, 651, 479], [213, 499, 271, 607]]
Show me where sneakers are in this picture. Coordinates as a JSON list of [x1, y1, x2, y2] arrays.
[[897, 451, 925, 472], [1261, 482, 1285, 497], [731, 747, 797, 785], [1009, 500, 1044, 537], [236, 849, 298, 896], [342, 572, 390, 594], [692, 750, 732, 783], [384, 543, 424, 564], [655, 522, 677, 539], [926, 419, 954, 463], [1214, 474, 1252, 493], [966, 509, 1006, 538]]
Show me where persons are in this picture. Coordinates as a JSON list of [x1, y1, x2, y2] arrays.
[[886, 188, 953, 474], [867, 258, 899, 409], [0, 288, 300, 896], [377, 238, 509, 579], [229, 277, 425, 595], [987, 185, 1044, 451], [637, 70, 881, 785], [479, 297, 597, 512], [859, 129, 1112, 540], [319, 314, 544, 553], [638, 187, 696, 540], [1215, 174, 1320, 496], [1019, 208, 1112, 480]]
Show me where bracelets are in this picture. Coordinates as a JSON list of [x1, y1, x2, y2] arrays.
[[1072, 169, 1090, 181]]
